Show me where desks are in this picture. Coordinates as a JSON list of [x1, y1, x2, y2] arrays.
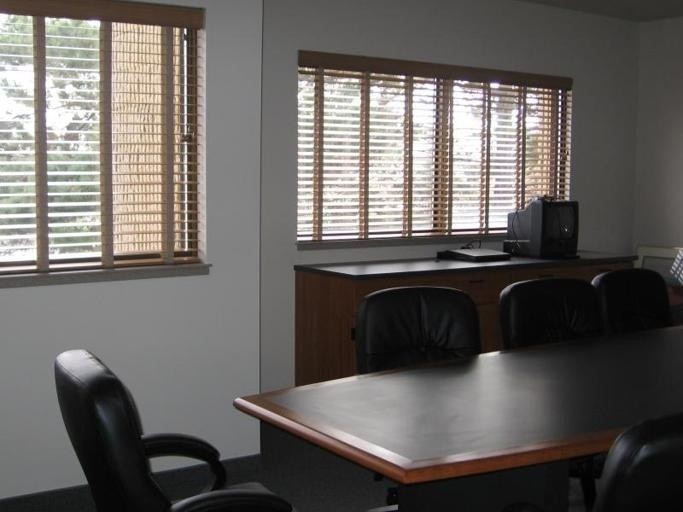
[[229, 324, 681, 485]]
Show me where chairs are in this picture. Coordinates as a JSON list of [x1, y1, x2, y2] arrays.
[[591, 266, 672, 330], [348, 283, 487, 505], [53, 348, 294, 511], [494, 275, 604, 353], [581, 417, 682, 510]]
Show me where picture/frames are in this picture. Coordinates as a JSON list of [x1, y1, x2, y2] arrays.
[[633, 243, 682, 287]]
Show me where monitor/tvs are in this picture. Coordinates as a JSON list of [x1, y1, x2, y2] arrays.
[[504, 200, 580, 257]]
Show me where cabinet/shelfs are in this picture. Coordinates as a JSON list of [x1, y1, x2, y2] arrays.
[[295, 248, 639, 387]]
